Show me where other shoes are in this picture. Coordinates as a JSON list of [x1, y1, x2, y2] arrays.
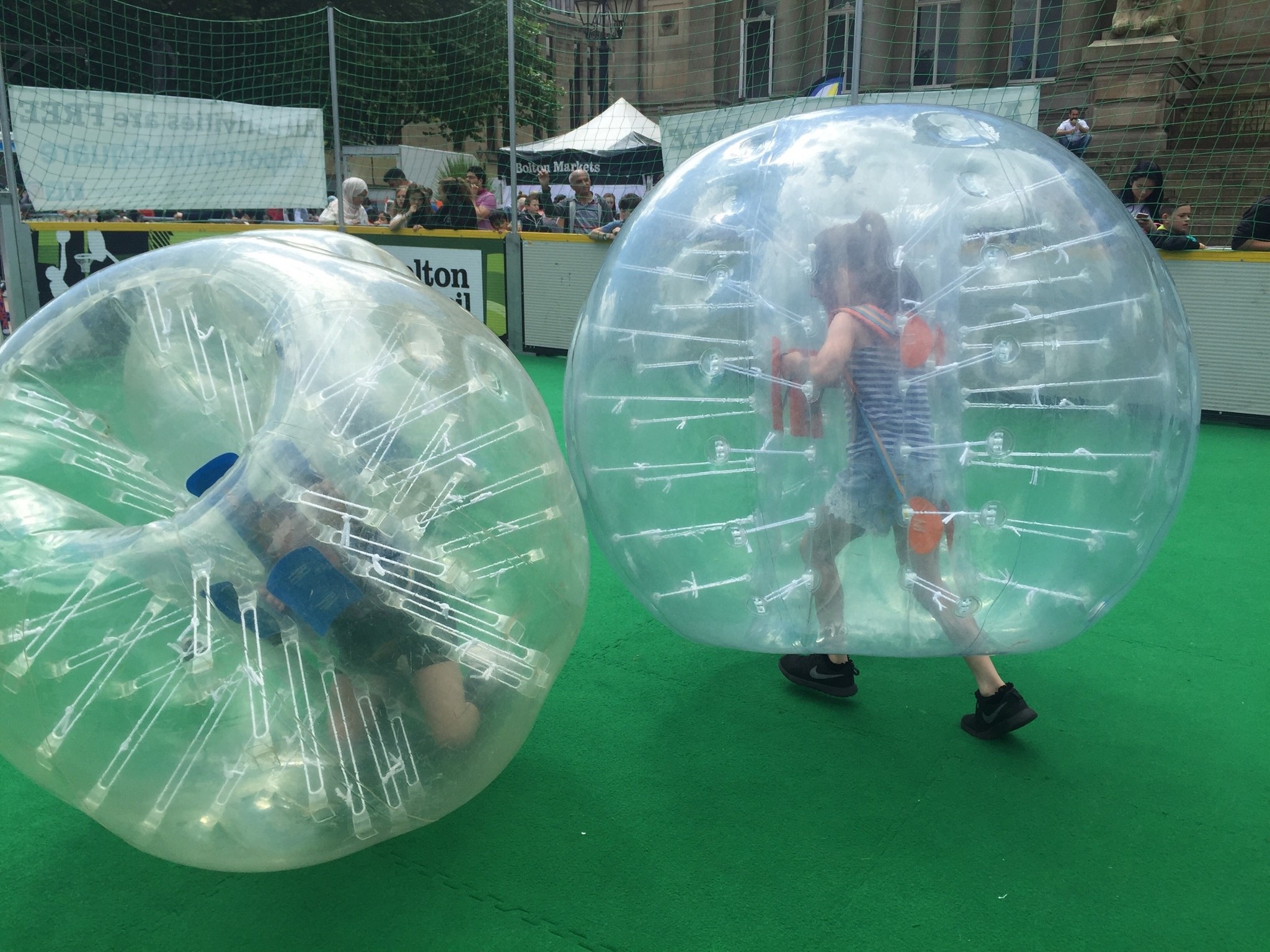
[[1076, 154, 1084, 159]]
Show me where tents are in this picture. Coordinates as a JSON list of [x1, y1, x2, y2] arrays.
[[497, 97, 664, 219]]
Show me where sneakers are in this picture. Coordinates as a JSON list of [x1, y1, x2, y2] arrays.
[[779, 650, 860, 698], [961, 682, 1038, 740]]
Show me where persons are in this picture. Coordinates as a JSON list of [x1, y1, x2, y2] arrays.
[[1116, 160, 1167, 242], [260, 480, 514, 757], [1, 164, 643, 242], [1152, 197, 1209, 251], [779, 213, 1036, 740], [1055, 107, 1092, 159], [1232, 196, 1270, 252]]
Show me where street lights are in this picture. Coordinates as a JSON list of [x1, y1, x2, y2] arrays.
[[574, 0, 632, 116]]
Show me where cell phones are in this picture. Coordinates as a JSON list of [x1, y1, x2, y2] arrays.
[[1136, 212, 1149, 218]]
